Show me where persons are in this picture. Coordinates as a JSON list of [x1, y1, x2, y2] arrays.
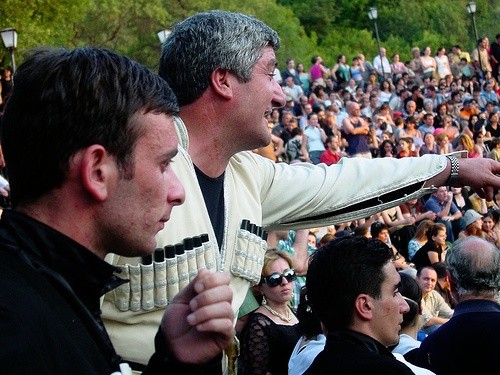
[[100, 9, 499, 375], [234, 32, 500, 375], [1, 46, 234, 375], [0, 64, 18, 210]]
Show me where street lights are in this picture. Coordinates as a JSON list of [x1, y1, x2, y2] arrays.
[[466, 2, 482, 74], [0, 27, 18, 83], [156, 29, 171, 45], [366, 8, 385, 80]]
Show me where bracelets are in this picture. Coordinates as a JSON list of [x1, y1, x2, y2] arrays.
[[446, 154, 459, 185]]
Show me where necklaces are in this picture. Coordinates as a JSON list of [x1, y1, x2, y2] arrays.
[[263, 304, 291, 322]]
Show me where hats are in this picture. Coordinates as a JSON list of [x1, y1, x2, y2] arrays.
[[461, 210, 483, 227]]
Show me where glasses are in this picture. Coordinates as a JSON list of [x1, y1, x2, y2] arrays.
[[263, 268, 296, 289]]
